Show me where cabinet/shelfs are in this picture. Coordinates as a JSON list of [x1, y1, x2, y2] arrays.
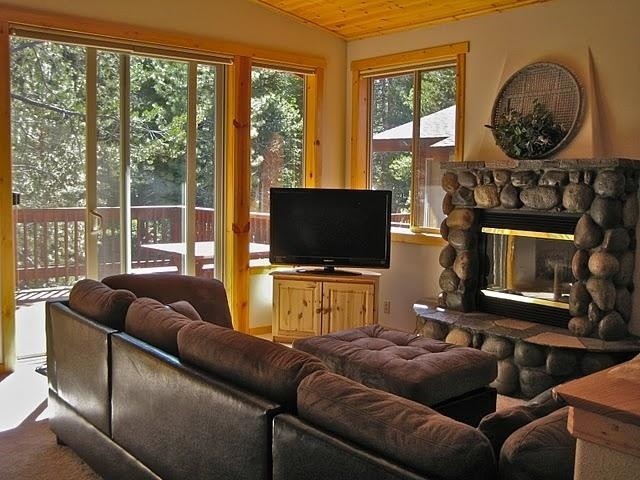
[[272, 273, 379, 344]]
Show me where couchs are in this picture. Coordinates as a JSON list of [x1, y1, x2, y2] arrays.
[[45, 272, 576, 480]]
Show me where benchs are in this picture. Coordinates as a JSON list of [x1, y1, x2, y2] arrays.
[[293, 323, 498, 428]]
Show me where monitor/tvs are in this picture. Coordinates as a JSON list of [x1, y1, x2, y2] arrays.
[[269, 187, 392, 276]]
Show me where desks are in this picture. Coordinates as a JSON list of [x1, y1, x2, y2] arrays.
[[140, 241, 270, 276]]
